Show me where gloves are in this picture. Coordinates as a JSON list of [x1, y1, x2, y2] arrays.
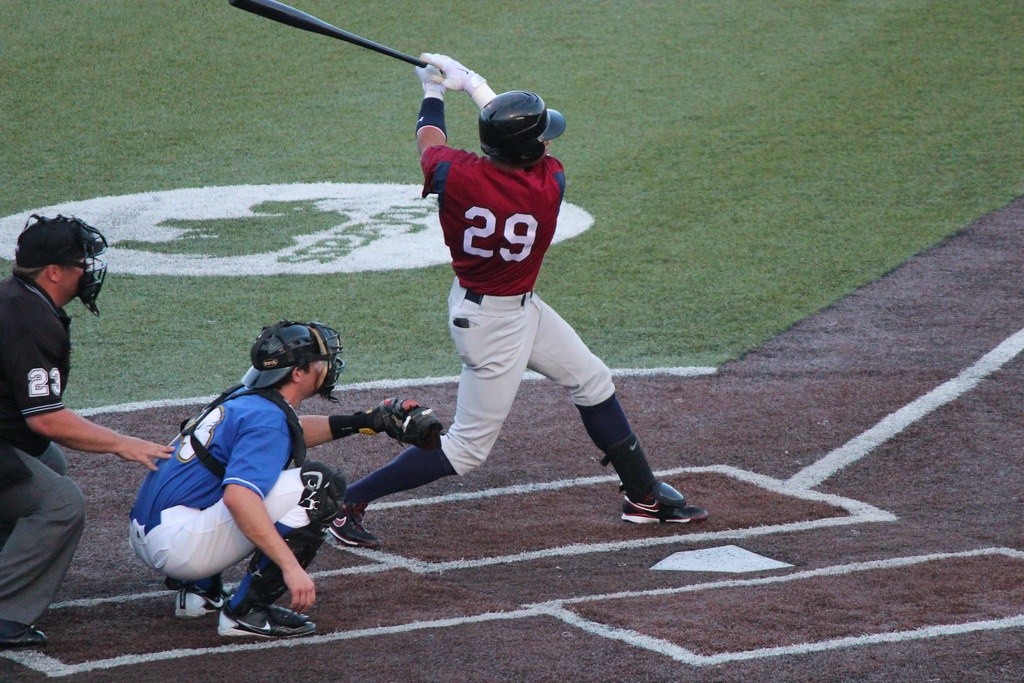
[[415, 53, 487, 98]]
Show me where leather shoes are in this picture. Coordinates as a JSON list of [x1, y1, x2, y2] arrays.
[[1, 624, 47, 646]]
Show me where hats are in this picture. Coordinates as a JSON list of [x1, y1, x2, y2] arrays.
[[16, 222, 103, 268]]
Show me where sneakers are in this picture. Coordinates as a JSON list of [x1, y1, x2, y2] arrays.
[[218, 600, 317, 638], [174, 583, 237, 620], [327, 502, 384, 549], [620, 482, 709, 524]]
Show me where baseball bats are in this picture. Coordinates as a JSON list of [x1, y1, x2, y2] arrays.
[[227, 1, 443, 74]]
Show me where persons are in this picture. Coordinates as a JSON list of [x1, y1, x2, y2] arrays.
[[0, 215, 175, 644], [323, 51, 709, 549], [129, 322, 442, 638]]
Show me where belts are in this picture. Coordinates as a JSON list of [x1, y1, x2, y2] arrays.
[[465, 290, 533, 307]]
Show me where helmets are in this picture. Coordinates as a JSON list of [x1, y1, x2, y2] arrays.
[[240, 321, 318, 388], [479, 90, 566, 172]]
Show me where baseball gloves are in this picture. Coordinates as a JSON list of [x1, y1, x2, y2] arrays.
[[382, 395, 444, 450]]
